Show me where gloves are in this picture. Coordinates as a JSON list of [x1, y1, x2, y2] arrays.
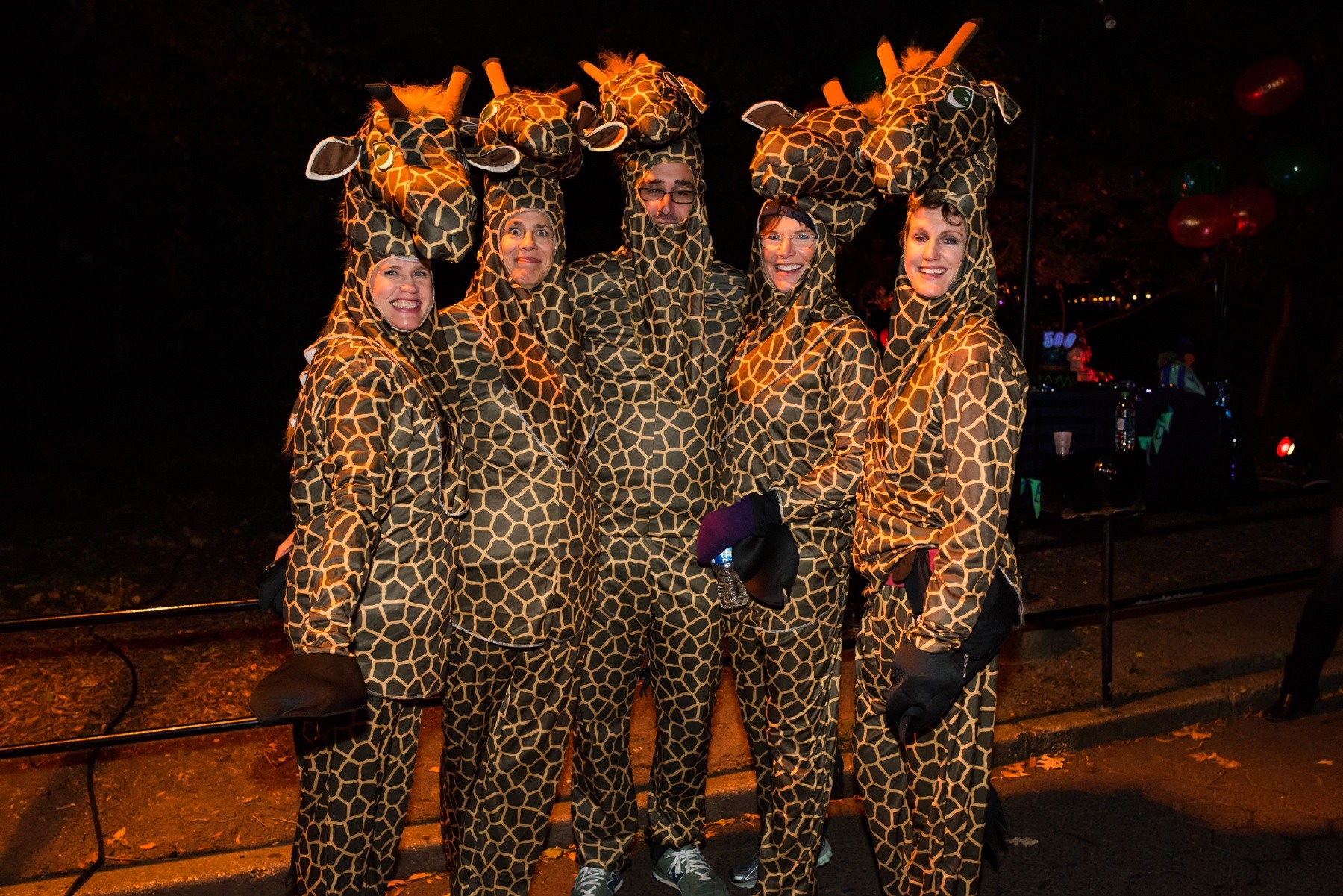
[[886, 640, 963, 744], [249, 653, 368, 722], [697, 489, 783, 569]]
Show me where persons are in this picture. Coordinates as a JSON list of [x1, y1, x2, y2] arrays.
[[694, 179, 878, 896], [560, 143, 743, 894], [845, 181, 1029, 894], [431, 177, 573, 894], [279, 239, 452, 896]]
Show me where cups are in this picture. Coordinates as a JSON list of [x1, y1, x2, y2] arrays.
[[711, 546, 749, 607], [1054, 432, 1072, 456]]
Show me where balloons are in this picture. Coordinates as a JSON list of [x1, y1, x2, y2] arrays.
[[1234, 49, 1305, 117], [1225, 184, 1276, 238], [1170, 192, 1235, 248]]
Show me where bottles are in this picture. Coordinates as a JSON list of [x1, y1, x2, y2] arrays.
[[1115, 392, 1135, 451]]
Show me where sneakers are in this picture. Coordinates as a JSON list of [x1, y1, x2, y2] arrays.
[[570, 865, 624, 896], [730, 838, 833, 888], [653, 843, 730, 896]]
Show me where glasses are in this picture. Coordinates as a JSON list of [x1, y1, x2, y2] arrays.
[[639, 188, 697, 204], [758, 232, 817, 248]]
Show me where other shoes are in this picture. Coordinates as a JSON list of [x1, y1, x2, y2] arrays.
[[1262, 692, 1317, 722]]
